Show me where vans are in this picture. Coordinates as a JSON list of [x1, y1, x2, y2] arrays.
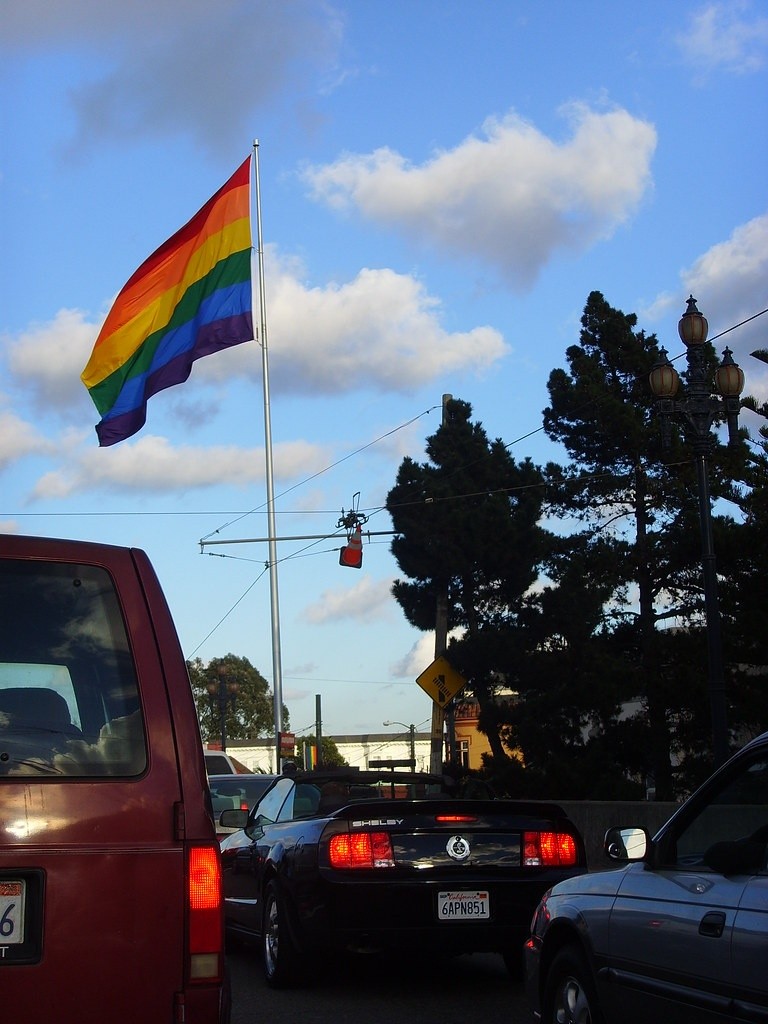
[[0, 535, 234, 1023], [199, 752, 251, 814]]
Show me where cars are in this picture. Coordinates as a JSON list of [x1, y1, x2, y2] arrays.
[[523, 731, 768, 1023], [206, 774, 281, 842]]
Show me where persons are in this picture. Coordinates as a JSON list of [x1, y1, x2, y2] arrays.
[[704, 824, 768, 872]]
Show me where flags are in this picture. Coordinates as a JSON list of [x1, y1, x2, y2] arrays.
[[80, 153, 256, 450]]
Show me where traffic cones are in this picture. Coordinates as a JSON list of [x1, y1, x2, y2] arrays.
[[339, 522, 364, 569]]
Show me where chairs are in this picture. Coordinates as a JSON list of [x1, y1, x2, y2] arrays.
[[0, 688, 83, 774], [212, 798, 234, 820]]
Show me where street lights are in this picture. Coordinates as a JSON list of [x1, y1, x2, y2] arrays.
[[382, 721, 415, 773], [205, 659, 239, 753]]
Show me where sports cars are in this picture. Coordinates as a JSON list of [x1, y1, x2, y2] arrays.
[[218, 773, 590, 992]]
